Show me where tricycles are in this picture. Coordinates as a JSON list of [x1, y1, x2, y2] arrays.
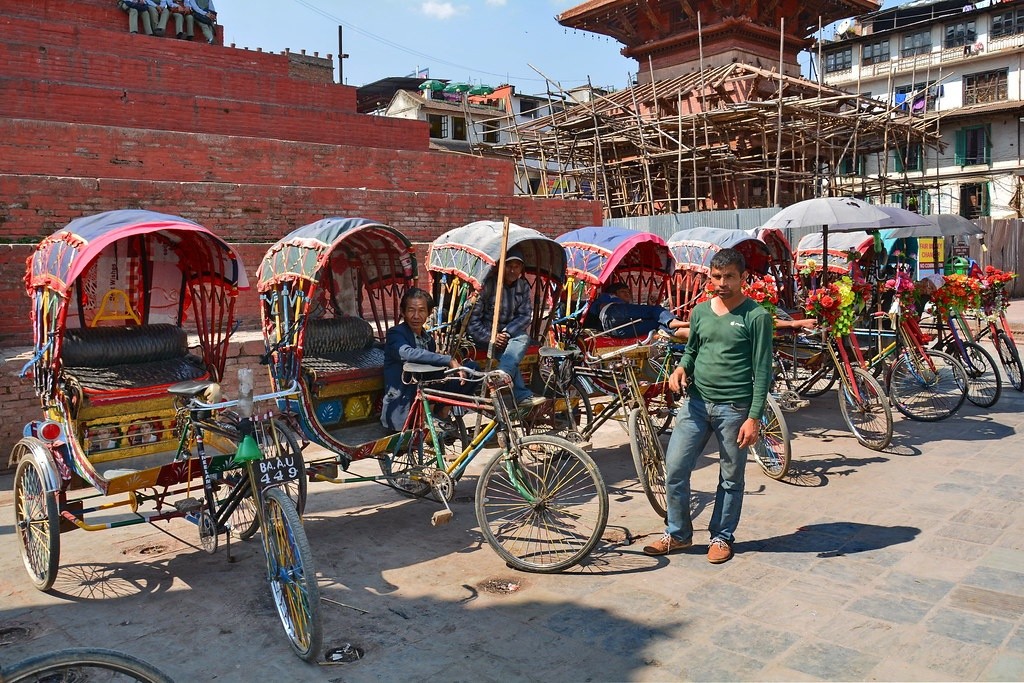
[[244, 216, 611, 575], [404, 195, 1024, 520], [7, 209, 328, 663]]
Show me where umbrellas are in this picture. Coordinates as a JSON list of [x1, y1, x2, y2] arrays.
[[418, 80, 495, 96]]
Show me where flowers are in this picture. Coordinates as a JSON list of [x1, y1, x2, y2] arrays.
[[743, 265, 1017, 338]]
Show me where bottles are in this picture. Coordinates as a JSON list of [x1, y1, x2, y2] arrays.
[[238, 368, 254, 420]]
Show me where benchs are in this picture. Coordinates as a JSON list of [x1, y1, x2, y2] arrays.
[[270, 316, 387, 397], [436, 300, 538, 371], [52, 321, 210, 422]]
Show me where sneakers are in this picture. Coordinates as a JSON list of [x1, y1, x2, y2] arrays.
[[491, 369, 514, 391], [643, 532, 693, 555], [517, 394, 546, 406], [707, 537, 732, 562]]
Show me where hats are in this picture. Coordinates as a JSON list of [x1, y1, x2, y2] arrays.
[[505, 248, 524, 262]]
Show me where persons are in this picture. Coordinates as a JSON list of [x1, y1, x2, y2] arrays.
[[458, 248, 546, 409], [590, 283, 691, 339], [380, 286, 482, 432], [118, 0, 217, 44], [760, 298, 819, 345], [643, 248, 773, 564], [881, 274, 945, 330]]
